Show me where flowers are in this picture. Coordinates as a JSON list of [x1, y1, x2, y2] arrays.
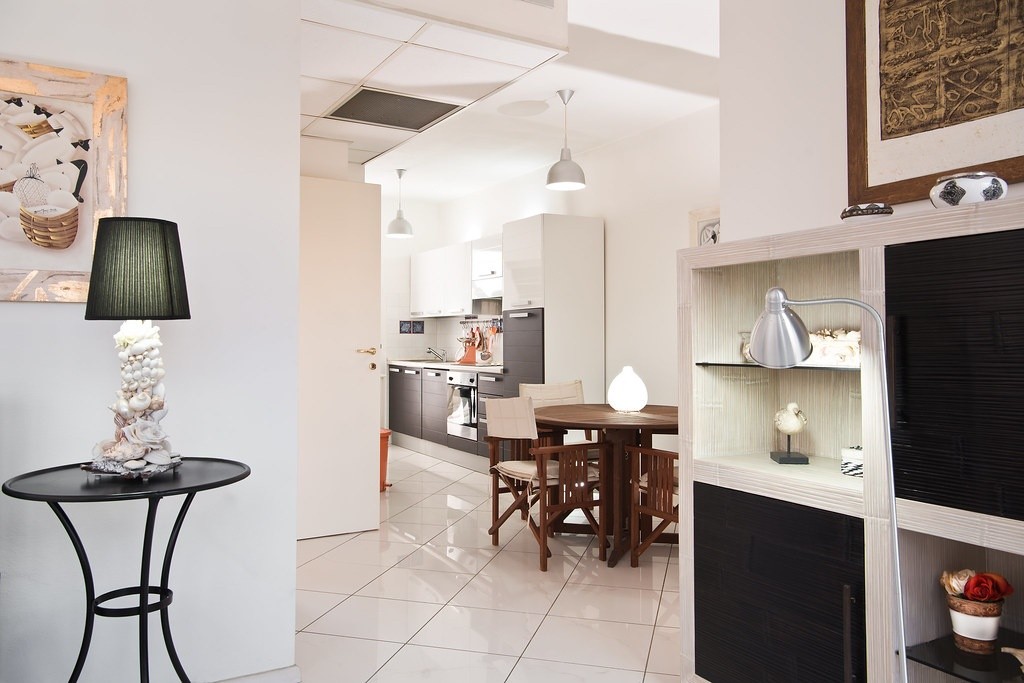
[[939, 568, 1013, 603]]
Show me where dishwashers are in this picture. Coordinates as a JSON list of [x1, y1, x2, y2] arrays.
[[446, 371, 478, 442]]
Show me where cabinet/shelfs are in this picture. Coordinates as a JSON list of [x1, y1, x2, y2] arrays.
[[410, 233, 503, 318], [676, 196, 1023, 683], [387, 364, 503, 476], [502, 213, 605, 446]]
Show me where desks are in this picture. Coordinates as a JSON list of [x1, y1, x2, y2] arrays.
[[533, 404, 678, 569], [2, 457, 251, 683]]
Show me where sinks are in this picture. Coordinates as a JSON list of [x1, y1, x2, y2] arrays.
[[397, 360, 441, 362]]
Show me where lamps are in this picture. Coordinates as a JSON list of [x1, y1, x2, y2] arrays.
[[81, 217, 191, 485], [385, 169, 412, 238], [607, 366, 648, 413], [544, 89, 585, 191], [748, 287, 908, 683]]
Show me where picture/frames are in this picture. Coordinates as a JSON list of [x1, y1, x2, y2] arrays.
[[0, 57, 128, 302], [845, 0, 1024, 207]]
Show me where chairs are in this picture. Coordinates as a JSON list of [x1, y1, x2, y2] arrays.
[[485, 380, 679, 572]]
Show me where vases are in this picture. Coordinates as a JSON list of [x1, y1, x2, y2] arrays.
[[947, 594, 1004, 654]]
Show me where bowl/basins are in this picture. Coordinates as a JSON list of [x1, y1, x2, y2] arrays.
[[929, 171, 1009, 209], [841, 203, 894, 223]]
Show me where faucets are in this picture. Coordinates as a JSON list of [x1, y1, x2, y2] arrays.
[[426, 347, 448, 362]]
